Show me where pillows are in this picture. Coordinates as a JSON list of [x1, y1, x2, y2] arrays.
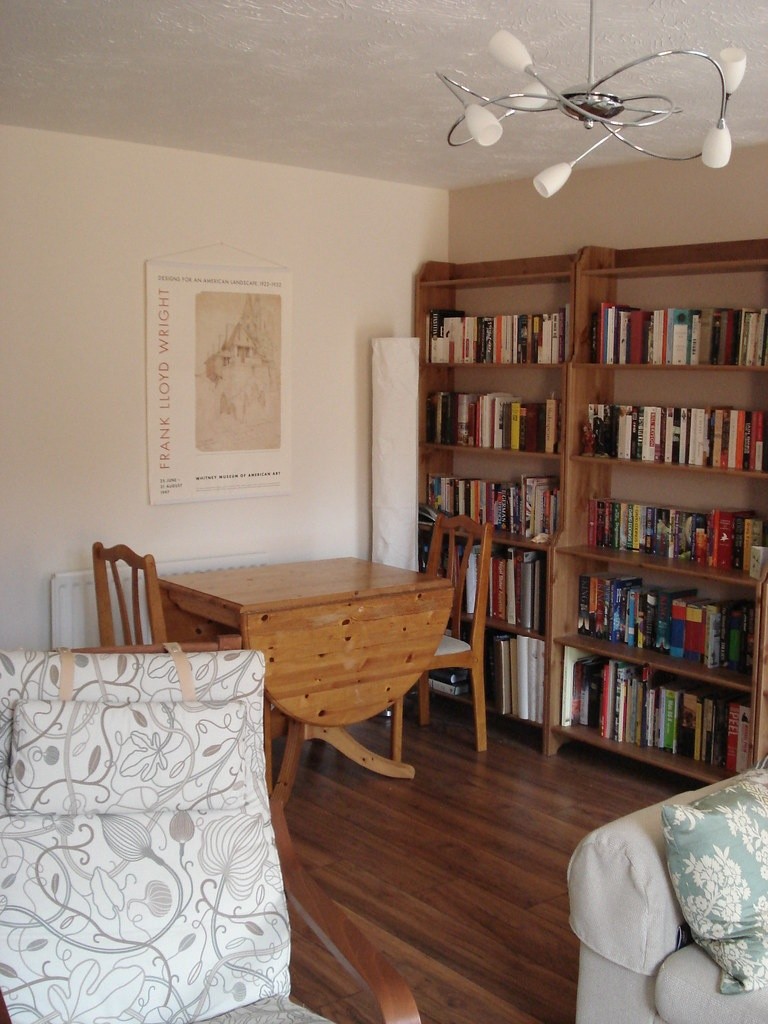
[[0, 811, 293, 1024], [660, 766, 768, 994], [5, 693, 252, 812]]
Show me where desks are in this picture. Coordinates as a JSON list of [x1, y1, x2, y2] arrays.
[[159, 556, 456, 811]]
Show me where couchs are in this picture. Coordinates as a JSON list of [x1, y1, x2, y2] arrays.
[[564, 769, 768, 1024]]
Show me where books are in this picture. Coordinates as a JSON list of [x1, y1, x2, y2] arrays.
[[423, 470, 764, 776], [427, 303, 768, 472]]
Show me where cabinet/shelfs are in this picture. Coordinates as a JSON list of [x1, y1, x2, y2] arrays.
[[413, 240, 768, 782]]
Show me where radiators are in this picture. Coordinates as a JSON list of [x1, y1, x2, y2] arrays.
[[47, 552, 270, 649]]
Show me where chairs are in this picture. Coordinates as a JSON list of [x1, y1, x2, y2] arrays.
[[386, 513, 496, 763], [0, 636, 425, 1024], [90, 542, 170, 645]]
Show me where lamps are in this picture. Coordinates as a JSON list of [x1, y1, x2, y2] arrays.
[[435, 0, 747, 198]]
